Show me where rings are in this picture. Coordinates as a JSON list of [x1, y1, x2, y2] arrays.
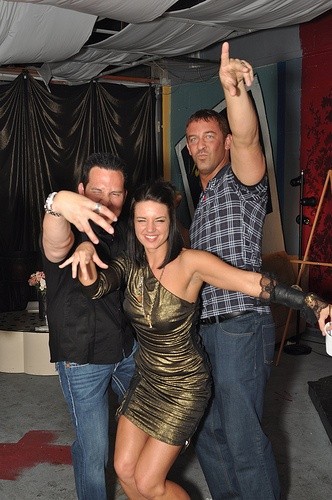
[[95, 203, 104, 213]]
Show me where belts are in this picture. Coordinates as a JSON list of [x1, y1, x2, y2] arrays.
[[197, 309, 254, 326]]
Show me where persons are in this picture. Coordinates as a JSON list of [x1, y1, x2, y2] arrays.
[[182, 42, 287, 500], [40, 154, 182, 500], [57, 181, 332, 500]]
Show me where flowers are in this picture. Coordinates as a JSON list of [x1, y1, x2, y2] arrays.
[[28, 272, 47, 296]]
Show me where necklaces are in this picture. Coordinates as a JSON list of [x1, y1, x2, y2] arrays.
[[140, 258, 163, 329]]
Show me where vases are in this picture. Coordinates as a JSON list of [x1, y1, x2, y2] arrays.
[[38, 291, 48, 321]]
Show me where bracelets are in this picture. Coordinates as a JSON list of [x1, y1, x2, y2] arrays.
[[44, 192, 61, 217]]
